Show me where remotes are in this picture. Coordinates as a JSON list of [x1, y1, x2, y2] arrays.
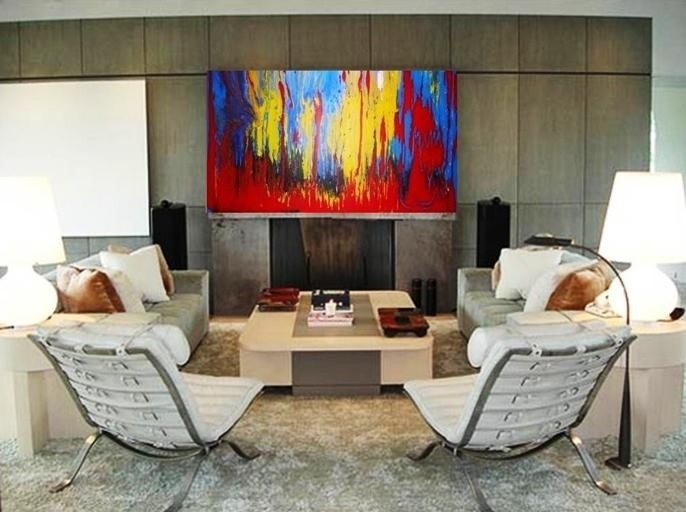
[[258, 304, 297, 313]]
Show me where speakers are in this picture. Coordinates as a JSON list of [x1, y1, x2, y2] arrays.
[[476, 196, 511, 268], [150, 200, 188, 270]]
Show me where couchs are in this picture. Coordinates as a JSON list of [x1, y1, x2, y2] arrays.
[[39, 253, 208, 368], [456, 250, 622, 341]]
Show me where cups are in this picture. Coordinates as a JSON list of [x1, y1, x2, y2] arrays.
[[325, 301, 336, 316]]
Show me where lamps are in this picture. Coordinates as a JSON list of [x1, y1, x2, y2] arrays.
[[523, 232, 635, 469], [597, 170, 685, 320], [0, 176, 66, 329]]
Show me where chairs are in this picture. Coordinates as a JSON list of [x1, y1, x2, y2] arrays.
[[402, 326, 637, 512], [28, 335, 264, 511]]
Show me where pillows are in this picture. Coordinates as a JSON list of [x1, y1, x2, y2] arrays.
[[55, 244, 175, 310], [492, 248, 610, 312]]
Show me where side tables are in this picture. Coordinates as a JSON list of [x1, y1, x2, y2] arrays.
[[579, 318, 686, 452]]
